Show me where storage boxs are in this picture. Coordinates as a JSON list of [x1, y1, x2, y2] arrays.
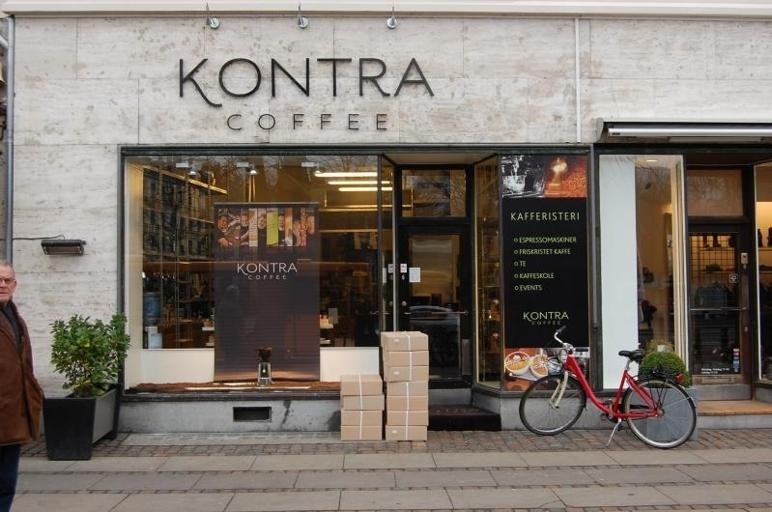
[[340, 330, 429, 442]]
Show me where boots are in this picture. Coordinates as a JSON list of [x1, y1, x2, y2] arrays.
[[703, 233, 710, 247], [713, 233, 721, 247]]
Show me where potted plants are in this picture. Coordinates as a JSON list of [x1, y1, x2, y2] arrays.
[[629, 351, 698, 442], [42, 311, 133, 460]]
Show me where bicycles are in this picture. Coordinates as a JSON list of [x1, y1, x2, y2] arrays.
[[519, 326, 696, 449]]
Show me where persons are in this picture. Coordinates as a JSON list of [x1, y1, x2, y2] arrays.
[[0, 261, 43, 512], [215, 211, 315, 250]]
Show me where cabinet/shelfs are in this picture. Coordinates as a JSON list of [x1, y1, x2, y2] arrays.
[[698, 247, 772, 274], [143, 165, 228, 348]]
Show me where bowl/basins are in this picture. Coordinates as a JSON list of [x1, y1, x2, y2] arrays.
[[504, 351, 549, 380]]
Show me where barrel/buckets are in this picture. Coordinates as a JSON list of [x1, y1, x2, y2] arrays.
[[143, 292, 161, 325]]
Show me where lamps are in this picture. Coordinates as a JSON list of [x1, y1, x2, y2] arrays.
[[205, 3, 220, 29], [296, 5, 309, 28], [313, 169, 393, 192], [387, 7, 397, 29], [41, 239, 84, 256]]
